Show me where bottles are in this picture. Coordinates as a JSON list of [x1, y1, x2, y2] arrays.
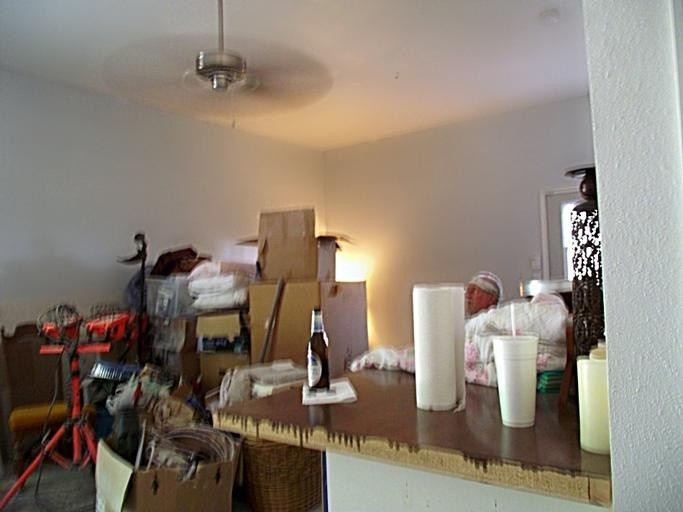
[[575, 344, 611, 457], [306, 304, 331, 394]]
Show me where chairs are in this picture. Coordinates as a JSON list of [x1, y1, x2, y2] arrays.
[[2, 324, 72, 493]]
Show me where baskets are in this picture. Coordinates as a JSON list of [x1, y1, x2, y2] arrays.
[[239, 438, 321, 512]]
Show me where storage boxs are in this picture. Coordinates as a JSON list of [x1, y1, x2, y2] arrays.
[[94, 208, 369, 512]]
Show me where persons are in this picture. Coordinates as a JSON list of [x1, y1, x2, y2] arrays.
[[465, 272, 504, 314]]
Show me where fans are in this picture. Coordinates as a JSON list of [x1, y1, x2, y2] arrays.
[[101, 0, 333, 122]]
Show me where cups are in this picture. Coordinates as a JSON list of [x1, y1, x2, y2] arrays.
[[491, 334, 540, 429]]
